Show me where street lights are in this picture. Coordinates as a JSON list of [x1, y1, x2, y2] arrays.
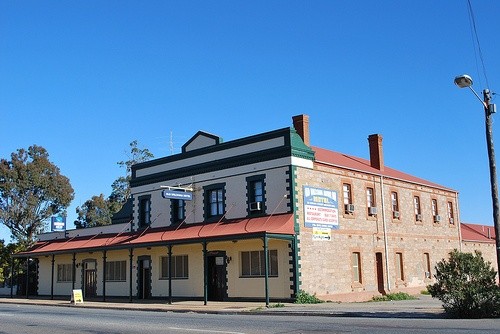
[[455, 74, 500, 281]]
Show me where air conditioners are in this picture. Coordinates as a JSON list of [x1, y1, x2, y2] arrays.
[[249, 202, 261, 211], [368, 207, 377, 215], [450, 218, 454, 224], [434, 215, 441, 221], [345, 204, 354, 212], [393, 211, 400, 217], [425, 272, 430, 278], [416, 214, 422, 220]]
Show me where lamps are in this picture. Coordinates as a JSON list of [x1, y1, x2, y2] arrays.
[[76, 262, 80, 268], [227, 256, 231, 264]]
